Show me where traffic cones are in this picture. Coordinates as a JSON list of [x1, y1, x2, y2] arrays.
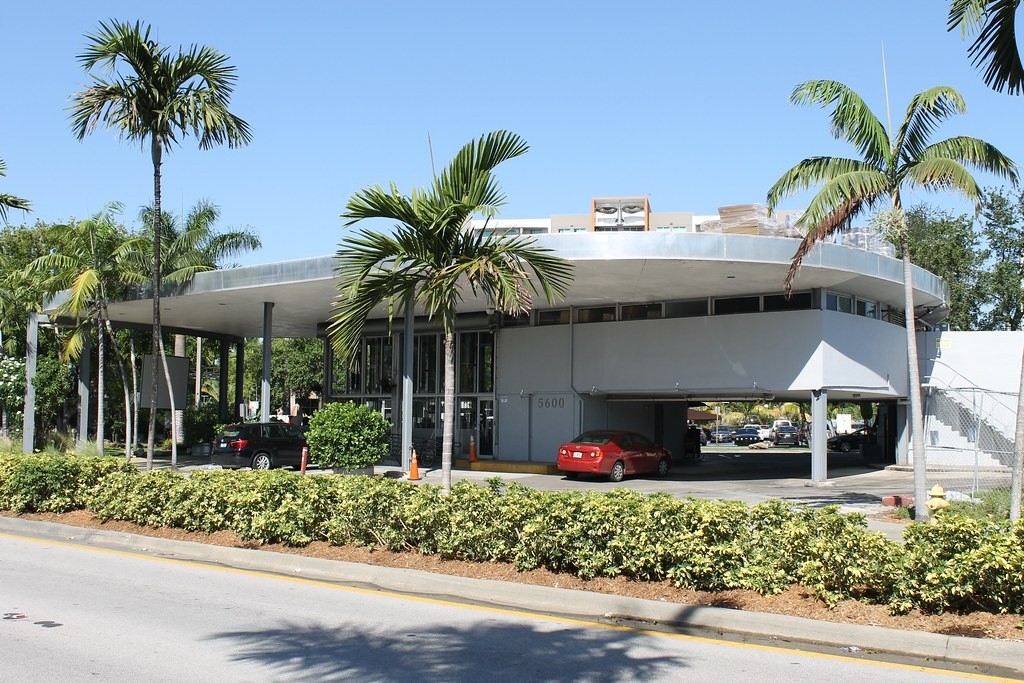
[[407, 448, 422, 480], [469, 434, 478, 462]]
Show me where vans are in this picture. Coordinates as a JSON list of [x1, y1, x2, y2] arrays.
[[769, 420, 792, 441]]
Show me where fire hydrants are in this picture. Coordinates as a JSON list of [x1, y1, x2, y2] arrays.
[[924, 483, 950, 516]]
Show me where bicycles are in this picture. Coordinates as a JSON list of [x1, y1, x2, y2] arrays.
[[397, 441, 435, 467]]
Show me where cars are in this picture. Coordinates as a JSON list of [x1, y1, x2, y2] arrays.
[[557, 430, 672, 482], [827, 428, 868, 453], [760, 426, 771, 438], [773, 426, 799, 446], [735, 428, 759, 445], [688, 427, 708, 446], [710, 425, 733, 442], [745, 424, 764, 440]]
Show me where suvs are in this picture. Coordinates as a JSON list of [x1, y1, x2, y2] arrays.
[[212, 422, 328, 472]]
